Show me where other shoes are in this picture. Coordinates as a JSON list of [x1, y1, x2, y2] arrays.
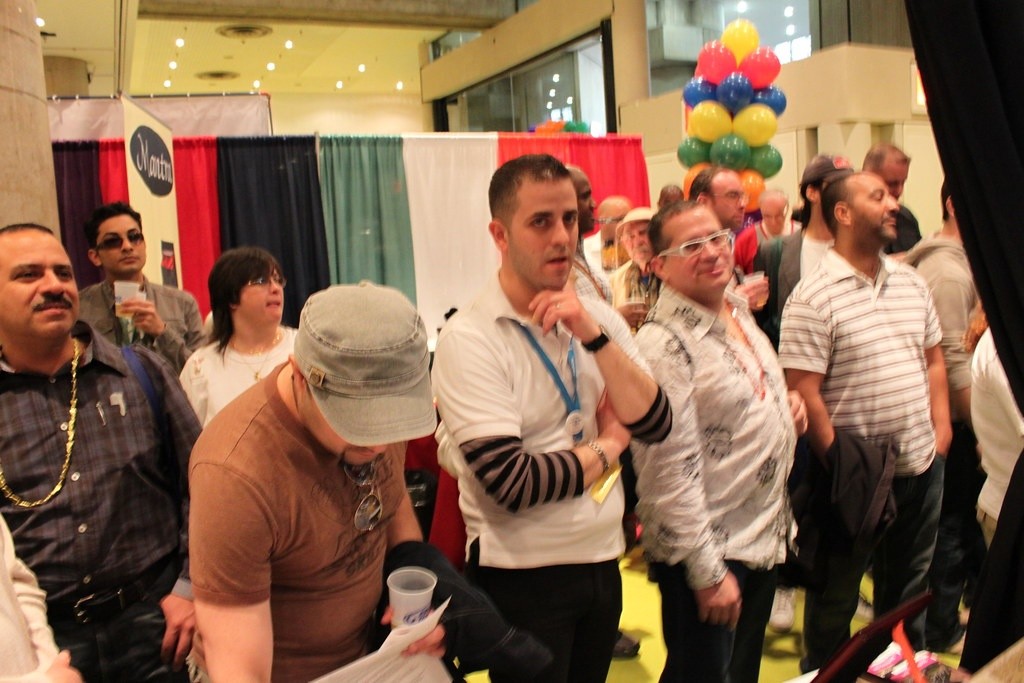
[[612, 634, 640, 657]]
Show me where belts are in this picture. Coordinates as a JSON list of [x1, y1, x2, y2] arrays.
[[47, 548, 180, 626]]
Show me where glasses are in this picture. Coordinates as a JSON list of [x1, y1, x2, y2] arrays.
[[656, 228, 731, 259], [709, 191, 744, 203], [95, 232, 144, 251], [243, 273, 287, 288], [343, 462, 383, 532]]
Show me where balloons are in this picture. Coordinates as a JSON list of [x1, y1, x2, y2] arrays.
[[679, 18, 787, 233], [529, 120, 592, 134]]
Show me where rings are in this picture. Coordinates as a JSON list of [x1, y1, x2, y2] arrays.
[[556, 303, 560, 308]]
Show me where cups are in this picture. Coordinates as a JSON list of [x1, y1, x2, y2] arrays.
[[114, 280, 139, 318], [744, 270, 767, 308], [386, 566, 438, 631]]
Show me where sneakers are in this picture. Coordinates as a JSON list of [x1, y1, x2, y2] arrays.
[[768, 587, 796, 633], [855, 598, 873, 622]]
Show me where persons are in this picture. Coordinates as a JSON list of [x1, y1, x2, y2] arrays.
[[0, 143, 1024, 682]]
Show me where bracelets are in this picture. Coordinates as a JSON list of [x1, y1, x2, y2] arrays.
[[586, 441, 610, 473]]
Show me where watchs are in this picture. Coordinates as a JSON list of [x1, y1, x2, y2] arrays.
[[581, 324, 611, 351]]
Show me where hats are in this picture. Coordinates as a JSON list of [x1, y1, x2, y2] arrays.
[[616, 208, 657, 237], [295, 280, 438, 447], [800, 152, 854, 182]]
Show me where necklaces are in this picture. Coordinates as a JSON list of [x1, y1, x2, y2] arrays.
[[0, 336, 79, 508]]
